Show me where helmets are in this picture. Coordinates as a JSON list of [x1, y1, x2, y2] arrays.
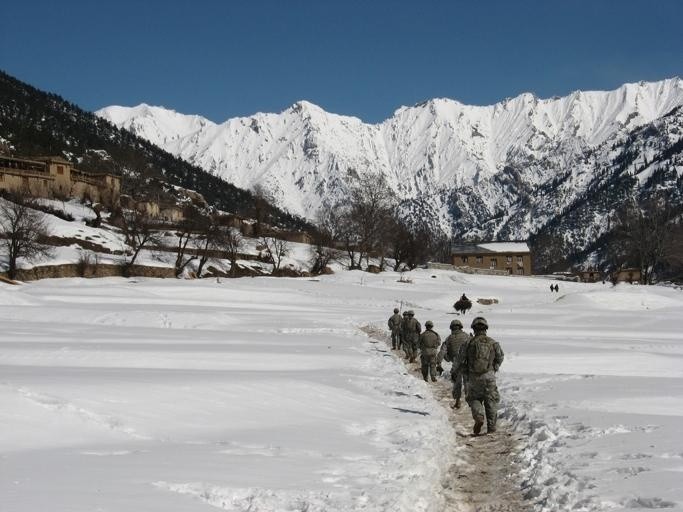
[[425, 321, 433, 327], [450, 320, 461, 325], [472, 317, 488, 329], [394, 308, 414, 315]]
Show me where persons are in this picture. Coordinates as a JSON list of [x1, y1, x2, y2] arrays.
[[555, 284, 559, 292], [388, 308, 402, 350], [417, 321, 441, 382], [399, 312, 409, 359], [550, 284, 554, 292], [436, 319, 473, 409], [449, 317, 505, 435], [460, 293, 468, 314], [405, 311, 421, 363]]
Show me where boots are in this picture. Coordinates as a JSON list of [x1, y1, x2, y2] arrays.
[[454, 398, 460, 408], [474, 415, 484, 433]]
